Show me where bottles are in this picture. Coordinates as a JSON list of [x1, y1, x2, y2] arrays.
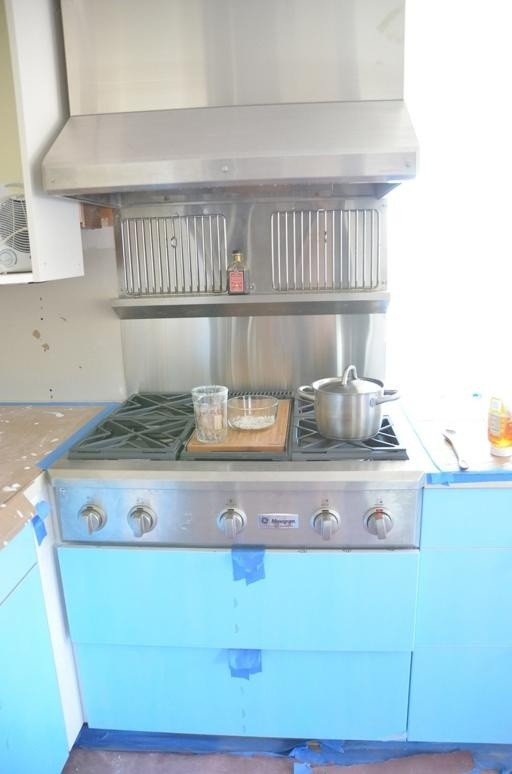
[[225, 249, 252, 298], [486, 396, 512, 458]]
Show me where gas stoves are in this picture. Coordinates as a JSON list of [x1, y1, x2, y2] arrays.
[[43, 391, 422, 553]]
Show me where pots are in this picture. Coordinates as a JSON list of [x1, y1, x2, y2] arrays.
[[296, 365, 400, 444]]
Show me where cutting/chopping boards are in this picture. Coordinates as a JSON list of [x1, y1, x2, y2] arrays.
[[183, 398, 293, 452]]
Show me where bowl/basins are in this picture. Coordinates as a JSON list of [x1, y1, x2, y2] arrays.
[[226, 396, 279, 431]]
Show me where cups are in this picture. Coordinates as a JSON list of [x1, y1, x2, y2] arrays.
[[189, 385, 228, 444]]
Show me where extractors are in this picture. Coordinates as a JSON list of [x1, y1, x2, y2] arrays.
[[38, 1, 420, 213]]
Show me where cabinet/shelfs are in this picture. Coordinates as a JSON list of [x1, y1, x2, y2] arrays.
[[0, 519, 70, 774], [57, 542, 420, 742], [406, 488, 512, 745]]
